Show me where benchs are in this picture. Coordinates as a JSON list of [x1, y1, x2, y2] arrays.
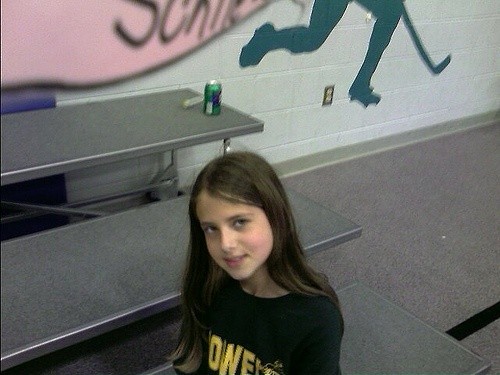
[[0, 86, 494, 375]]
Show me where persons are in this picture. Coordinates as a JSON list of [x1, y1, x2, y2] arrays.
[[159, 148, 346, 375]]
